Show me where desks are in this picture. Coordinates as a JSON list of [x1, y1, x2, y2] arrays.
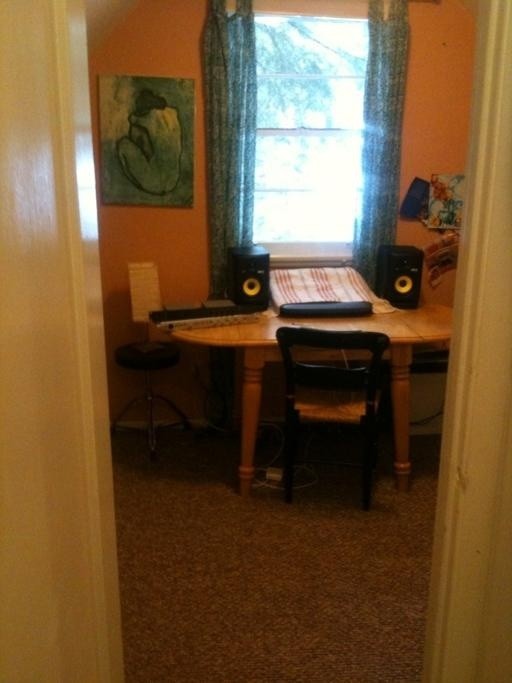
[[165, 300, 454, 498]]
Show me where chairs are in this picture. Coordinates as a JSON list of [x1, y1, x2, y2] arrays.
[[274, 325, 392, 512]]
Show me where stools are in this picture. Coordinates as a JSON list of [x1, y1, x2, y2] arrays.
[[110, 338, 195, 463]]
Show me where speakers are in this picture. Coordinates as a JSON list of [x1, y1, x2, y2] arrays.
[[378, 245, 424, 309], [227, 246, 270, 312]]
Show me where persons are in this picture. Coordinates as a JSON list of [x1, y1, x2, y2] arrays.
[[117, 89, 181, 193]]
